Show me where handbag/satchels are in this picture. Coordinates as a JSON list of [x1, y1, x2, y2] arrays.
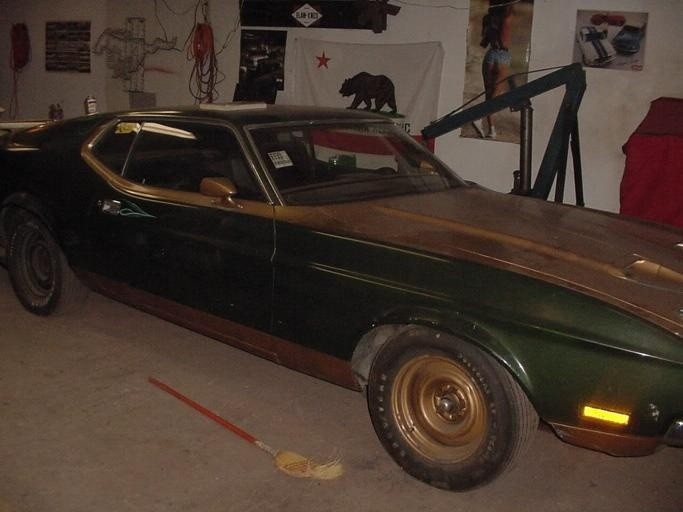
[[509, 98, 531, 112]]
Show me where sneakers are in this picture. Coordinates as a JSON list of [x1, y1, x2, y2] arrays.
[[471, 118, 485, 138], [486, 132, 496, 138]]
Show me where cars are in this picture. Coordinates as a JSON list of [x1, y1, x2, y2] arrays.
[[1, 104, 683, 491], [577, 25, 616, 66], [612, 21, 646, 54]]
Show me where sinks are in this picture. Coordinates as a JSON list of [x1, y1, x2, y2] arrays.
[[0, 120, 52, 130]]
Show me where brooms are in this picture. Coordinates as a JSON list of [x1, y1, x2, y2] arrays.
[[146, 375, 343, 483]]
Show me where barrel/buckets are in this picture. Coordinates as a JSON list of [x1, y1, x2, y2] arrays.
[[52, 99, 64, 122]]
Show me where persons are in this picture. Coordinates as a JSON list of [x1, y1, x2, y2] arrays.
[[471, 0, 514, 139]]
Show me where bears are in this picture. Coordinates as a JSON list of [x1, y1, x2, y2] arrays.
[[338, 71, 397, 114]]
